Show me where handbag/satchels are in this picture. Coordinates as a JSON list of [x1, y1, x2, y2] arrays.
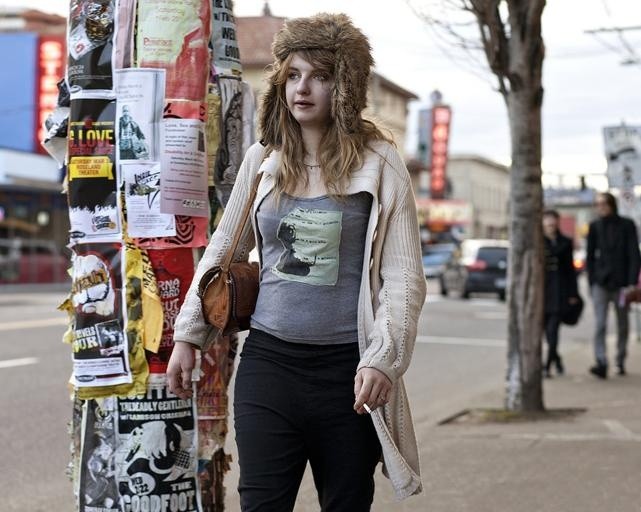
[[197, 262, 259, 338]]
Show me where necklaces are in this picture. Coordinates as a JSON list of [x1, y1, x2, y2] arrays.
[[303, 163, 324, 170]]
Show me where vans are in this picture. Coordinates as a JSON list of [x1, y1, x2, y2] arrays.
[[0, 236, 57, 266]]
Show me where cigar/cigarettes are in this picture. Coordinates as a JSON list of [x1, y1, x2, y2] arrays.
[[363, 403, 371, 414]]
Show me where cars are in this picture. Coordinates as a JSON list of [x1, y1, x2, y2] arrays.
[[435, 237, 509, 301], [420, 242, 461, 279]]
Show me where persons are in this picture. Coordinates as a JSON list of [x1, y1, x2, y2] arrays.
[[118, 105, 145, 159], [543, 210, 579, 377], [168, 11, 427, 511], [588, 193, 641, 378]]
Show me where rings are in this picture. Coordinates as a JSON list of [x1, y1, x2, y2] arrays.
[[378, 395, 385, 401]]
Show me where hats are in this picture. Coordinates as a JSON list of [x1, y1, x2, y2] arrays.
[[256, 12, 375, 144]]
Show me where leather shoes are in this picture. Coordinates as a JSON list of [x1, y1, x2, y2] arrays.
[[589, 361, 628, 381]]
